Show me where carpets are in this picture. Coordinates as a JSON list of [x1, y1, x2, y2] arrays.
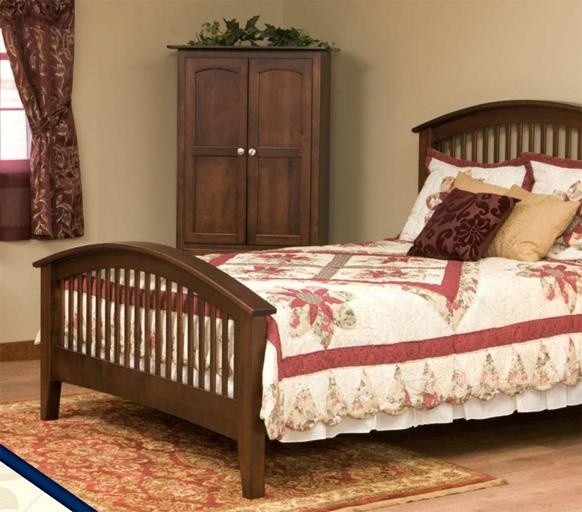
[[0, 389, 509, 511]]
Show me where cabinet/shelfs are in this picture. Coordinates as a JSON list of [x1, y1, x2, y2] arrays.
[[165, 38, 341, 260]]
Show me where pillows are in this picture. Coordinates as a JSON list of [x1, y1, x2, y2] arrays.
[[397, 145, 582, 263]]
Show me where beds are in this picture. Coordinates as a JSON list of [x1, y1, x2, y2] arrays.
[[26, 100, 581, 499]]
